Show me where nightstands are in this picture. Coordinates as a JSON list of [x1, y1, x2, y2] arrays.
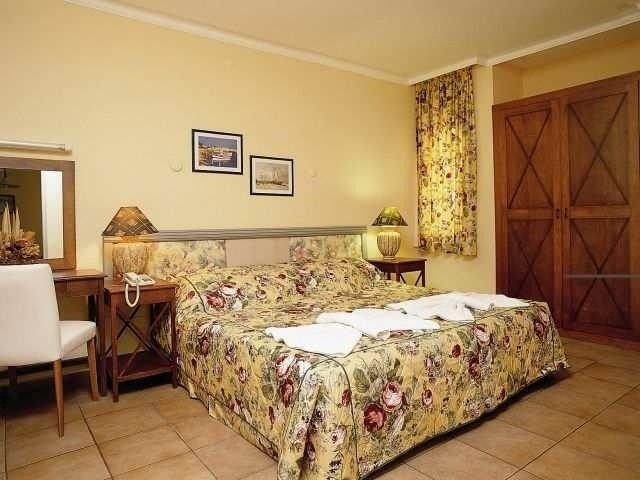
[[102, 280, 178, 404], [369, 256, 427, 287]]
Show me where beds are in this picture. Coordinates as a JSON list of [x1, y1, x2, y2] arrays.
[[103, 226, 569, 480]]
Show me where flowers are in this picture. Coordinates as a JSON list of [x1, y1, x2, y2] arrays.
[[0, 230, 43, 264]]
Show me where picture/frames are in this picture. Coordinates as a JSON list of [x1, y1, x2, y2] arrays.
[[189, 128, 245, 177], [0, 157, 77, 270], [249, 154, 293, 198]]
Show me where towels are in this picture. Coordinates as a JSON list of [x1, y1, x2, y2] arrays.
[[319, 303, 440, 339], [384, 296, 476, 323], [264, 322, 361, 356], [446, 289, 530, 311]]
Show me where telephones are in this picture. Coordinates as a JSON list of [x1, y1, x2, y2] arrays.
[[122, 271, 157, 286]]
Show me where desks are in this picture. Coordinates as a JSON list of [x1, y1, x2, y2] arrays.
[[54, 267, 110, 399]]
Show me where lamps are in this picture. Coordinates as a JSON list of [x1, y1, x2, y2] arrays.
[[371, 206, 406, 259], [103, 204, 158, 278]]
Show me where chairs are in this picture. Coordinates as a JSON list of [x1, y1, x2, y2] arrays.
[[0, 264, 100, 441]]
